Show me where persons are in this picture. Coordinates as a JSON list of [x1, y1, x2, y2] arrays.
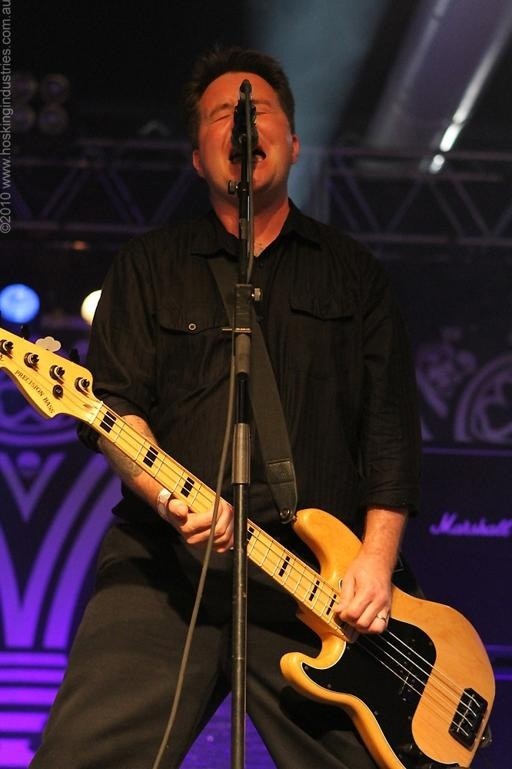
[[30, 41, 426, 763]]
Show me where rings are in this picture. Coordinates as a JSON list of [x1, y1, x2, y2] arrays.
[[374, 614, 388, 624]]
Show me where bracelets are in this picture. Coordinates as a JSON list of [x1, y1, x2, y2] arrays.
[[155, 485, 173, 522]]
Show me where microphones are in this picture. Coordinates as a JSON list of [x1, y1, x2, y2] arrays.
[[231, 83, 259, 153]]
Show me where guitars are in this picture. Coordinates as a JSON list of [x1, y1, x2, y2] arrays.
[[0, 329, 496, 768]]
